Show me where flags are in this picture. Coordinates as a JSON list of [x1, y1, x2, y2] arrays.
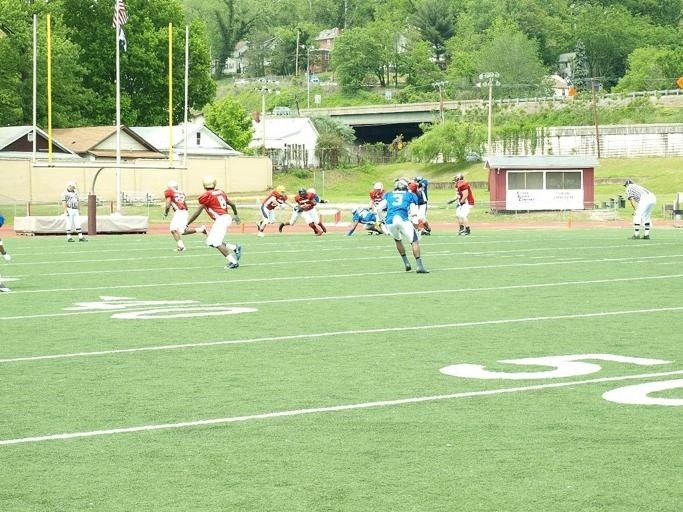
[[115, 25, 127, 52], [110, 0, 128, 27]]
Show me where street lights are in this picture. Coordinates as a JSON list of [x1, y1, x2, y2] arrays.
[[474, 69, 501, 157], [300, 44, 315, 109], [253, 84, 280, 156]]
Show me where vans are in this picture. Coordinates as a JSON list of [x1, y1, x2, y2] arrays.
[[272, 106, 289, 113]]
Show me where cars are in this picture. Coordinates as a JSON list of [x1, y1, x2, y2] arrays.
[[310, 78, 320, 85]]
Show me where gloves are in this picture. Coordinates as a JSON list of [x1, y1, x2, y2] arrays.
[[233, 215, 241, 224], [3, 252, 11, 261]]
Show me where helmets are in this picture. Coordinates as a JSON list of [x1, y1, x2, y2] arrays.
[[299, 188, 306, 196], [394, 181, 408, 190], [203, 176, 216, 189], [167, 181, 179, 190], [276, 186, 285, 196], [374, 183, 383, 193], [454, 174, 463, 182]]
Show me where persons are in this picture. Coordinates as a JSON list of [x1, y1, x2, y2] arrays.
[[622, 179, 657, 239], [0, 212, 12, 292], [161, 179, 208, 252], [184, 177, 243, 269], [347, 172, 476, 274], [253, 184, 330, 235], [61, 180, 89, 243]]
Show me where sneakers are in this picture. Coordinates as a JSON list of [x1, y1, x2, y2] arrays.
[[641, 235, 649, 239], [224, 262, 239, 270], [416, 267, 429, 273], [68, 234, 74, 242], [0, 282, 10, 291], [629, 235, 640, 239], [458, 229, 470, 236], [202, 225, 208, 235], [405, 264, 411, 271], [175, 246, 185, 252], [256, 221, 327, 236], [234, 244, 240, 260], [78, 236, 88, 242]]
